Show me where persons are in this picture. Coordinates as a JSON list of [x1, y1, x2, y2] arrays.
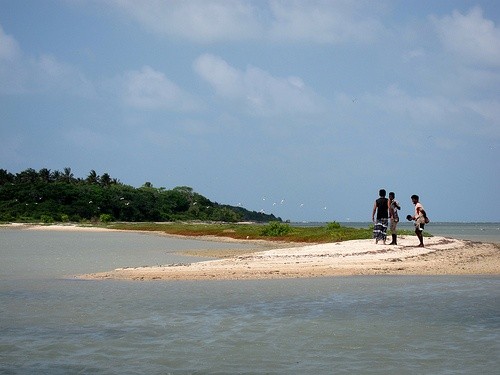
[[388, 192, 400, 245], [410, 194, 427, 248], [372, 189, 392, 246]]
